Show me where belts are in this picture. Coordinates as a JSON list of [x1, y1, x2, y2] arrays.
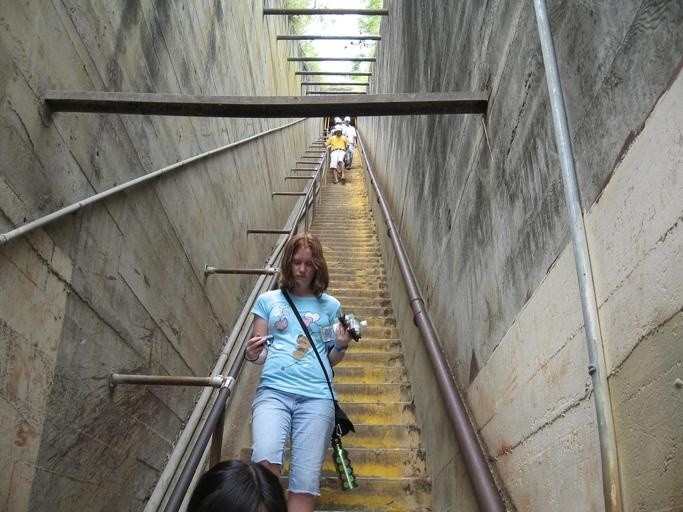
[[329, 148, 346, 153]]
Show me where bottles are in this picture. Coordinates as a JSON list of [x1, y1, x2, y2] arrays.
[[321, 320, 369, 343], [331, 438, 357, 492]]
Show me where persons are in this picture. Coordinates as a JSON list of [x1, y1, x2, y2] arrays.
[[181, 456, 290, 511], [323, 116, 358, 183], [243, 229, 355, 512]]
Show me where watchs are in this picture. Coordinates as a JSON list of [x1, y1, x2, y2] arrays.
[[333, 344, 349, 352]]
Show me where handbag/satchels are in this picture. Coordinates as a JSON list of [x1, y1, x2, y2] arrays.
[[331, 401, 355, 438]]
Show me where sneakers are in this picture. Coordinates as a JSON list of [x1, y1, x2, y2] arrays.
[[332, 163, 350, 184]]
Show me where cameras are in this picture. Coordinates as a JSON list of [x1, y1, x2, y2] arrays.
[[258, 335, 274, 347]]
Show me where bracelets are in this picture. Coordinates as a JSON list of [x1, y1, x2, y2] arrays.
[[243, 350, 260, 362]]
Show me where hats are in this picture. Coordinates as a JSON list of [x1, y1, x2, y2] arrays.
[[343, 116, 350, 122], [330, 116, 342, 133]]
